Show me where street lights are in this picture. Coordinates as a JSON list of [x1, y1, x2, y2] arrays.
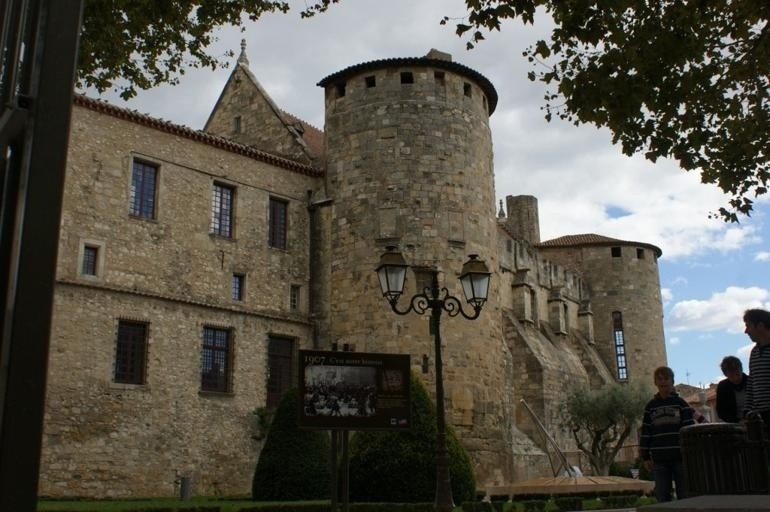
[[374, 245, 492, 512]]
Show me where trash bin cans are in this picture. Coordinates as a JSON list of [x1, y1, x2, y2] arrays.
[[679, 422, 751, 498]]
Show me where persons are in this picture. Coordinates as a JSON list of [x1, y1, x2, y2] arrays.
[[672, 385, 710, 425], [639, 367, 695, 502], [743, 308, 770, 461], [714, 355, 754, 421]]
